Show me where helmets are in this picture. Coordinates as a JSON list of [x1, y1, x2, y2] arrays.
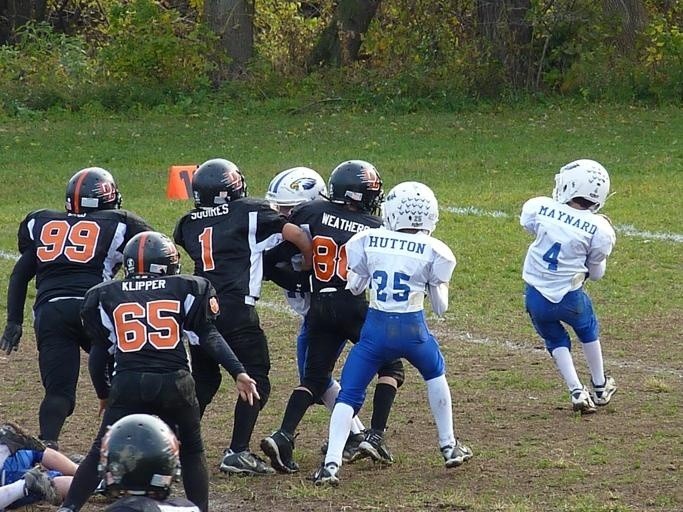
[[264, 165, 327, 212], [326, 158, 382, 213], [192, 158, 248, 210], [380, 181, 440, 236], [552, 158, 610, 214], [123, 229, 180, 277], [97, 412, 181, 496], [64, 166, 124, 215]]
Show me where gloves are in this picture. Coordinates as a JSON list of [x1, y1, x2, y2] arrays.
[[1, 322, 23, 356]]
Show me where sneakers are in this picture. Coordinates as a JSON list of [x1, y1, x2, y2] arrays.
[[590, 375, 617, 406], [0, 419, 47, 457], [20, 462, 63, 506], [568, 384, 597, 414], [218, 429, 396, 487], [439, 440, 473, 469]]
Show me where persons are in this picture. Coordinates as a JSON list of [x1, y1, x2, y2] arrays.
[[0, 158, 473, 512], [516, 158, 617, 415]]
